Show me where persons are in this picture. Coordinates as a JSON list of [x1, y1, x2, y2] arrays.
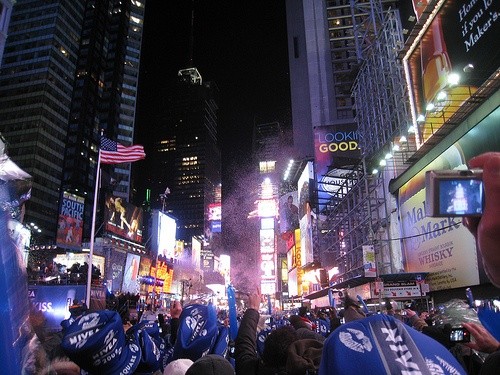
[[50, 167, 500, 256], [0, 255, 500, 375]]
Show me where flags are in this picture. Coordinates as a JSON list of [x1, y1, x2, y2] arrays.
[[98, 136, 145, 164]]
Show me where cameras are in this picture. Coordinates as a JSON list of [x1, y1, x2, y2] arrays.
[[425, 168, 485, 218], [446, 328, 471, 343]]
